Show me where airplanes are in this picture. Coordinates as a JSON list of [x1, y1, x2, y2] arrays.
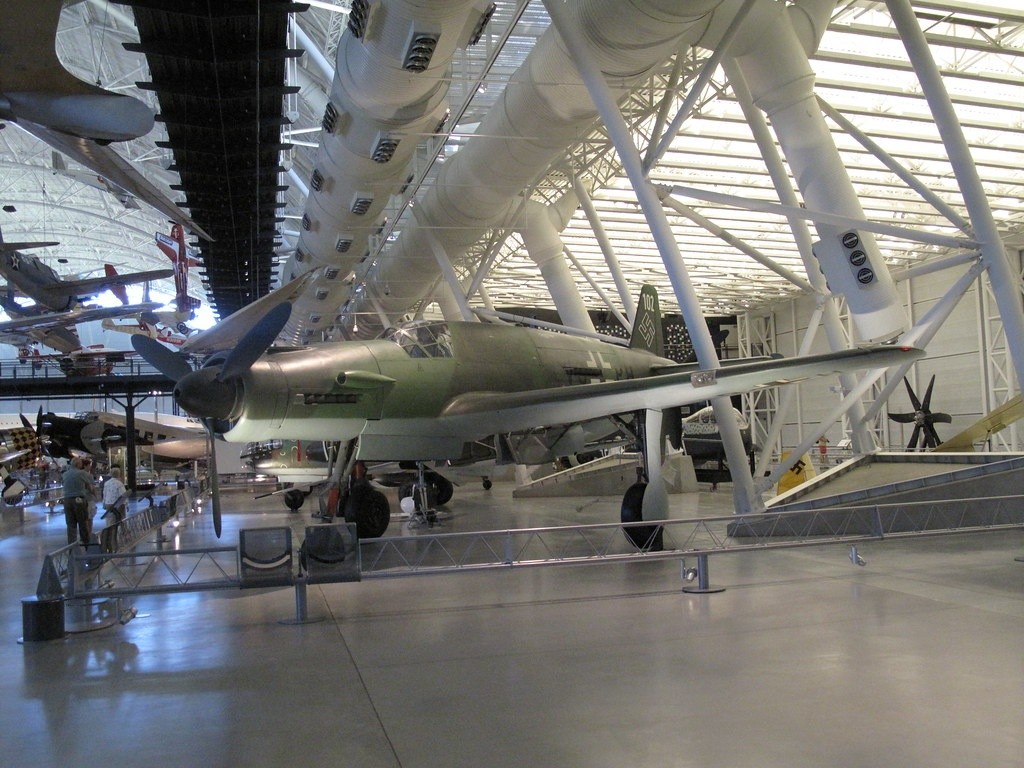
[[2, 0, 217, 509], [681, 403, 749, 459], [122, 300, 926, 549]]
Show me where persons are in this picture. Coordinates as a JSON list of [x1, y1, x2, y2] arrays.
[[76, 459, 99, 534], [62, 456, 94, 555], [100, 467, 128, 554]]
